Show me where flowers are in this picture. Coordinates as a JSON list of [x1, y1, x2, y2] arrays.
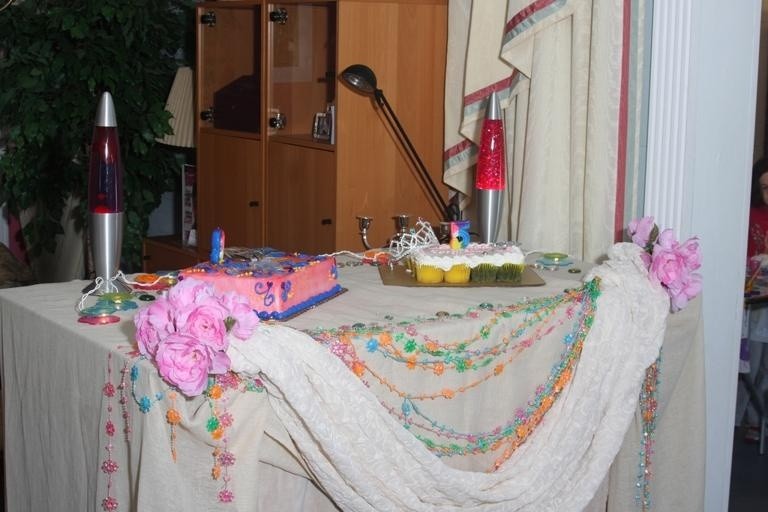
[[132, 277, 261, 397], [628, 214, 704, 312]]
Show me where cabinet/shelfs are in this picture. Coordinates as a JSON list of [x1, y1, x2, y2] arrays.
[[143, 0, 448, 275]]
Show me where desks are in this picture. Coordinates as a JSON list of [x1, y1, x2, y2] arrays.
[[1, 242, 708, 512]]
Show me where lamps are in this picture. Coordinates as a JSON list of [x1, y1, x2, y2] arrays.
[[339, 64, 461, 242]]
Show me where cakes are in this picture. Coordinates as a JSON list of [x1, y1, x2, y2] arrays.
[[178, 228, 341, 321], [407, 242, 524, 283]]
